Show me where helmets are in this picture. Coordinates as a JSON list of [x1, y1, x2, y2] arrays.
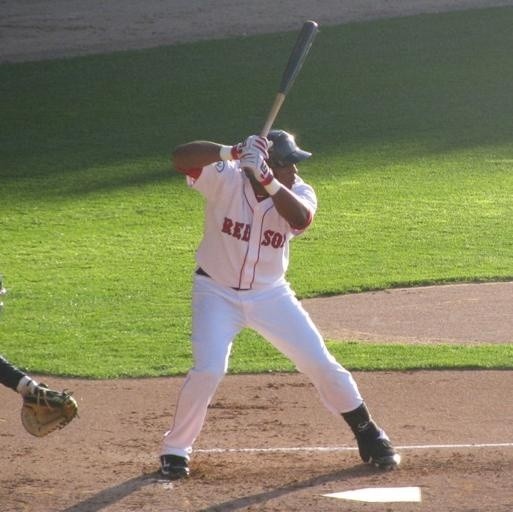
[[254, 130, 312, 169]]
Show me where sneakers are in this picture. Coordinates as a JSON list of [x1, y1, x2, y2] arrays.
[[160, 456, 190, 480], [354, 420, 399, 471]]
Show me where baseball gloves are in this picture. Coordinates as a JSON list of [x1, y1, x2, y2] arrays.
[[22, 382, 79, 437]]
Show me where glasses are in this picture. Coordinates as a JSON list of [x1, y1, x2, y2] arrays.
[[272, 157, 295, 167]]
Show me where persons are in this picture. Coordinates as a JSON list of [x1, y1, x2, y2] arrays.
[[160, 130, 402, 478], [1, 358, 77, 437]]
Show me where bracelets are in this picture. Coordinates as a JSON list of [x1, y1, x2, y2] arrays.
[[264, 178, 282, 196], [220, 145, 233, 163]]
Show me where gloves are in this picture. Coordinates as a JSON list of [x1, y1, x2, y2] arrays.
[[231, 135, 269, 162], [240, 151, 274, 185]]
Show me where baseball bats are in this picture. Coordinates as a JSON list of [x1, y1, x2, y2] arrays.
[[243, 19, 319, 177]]
